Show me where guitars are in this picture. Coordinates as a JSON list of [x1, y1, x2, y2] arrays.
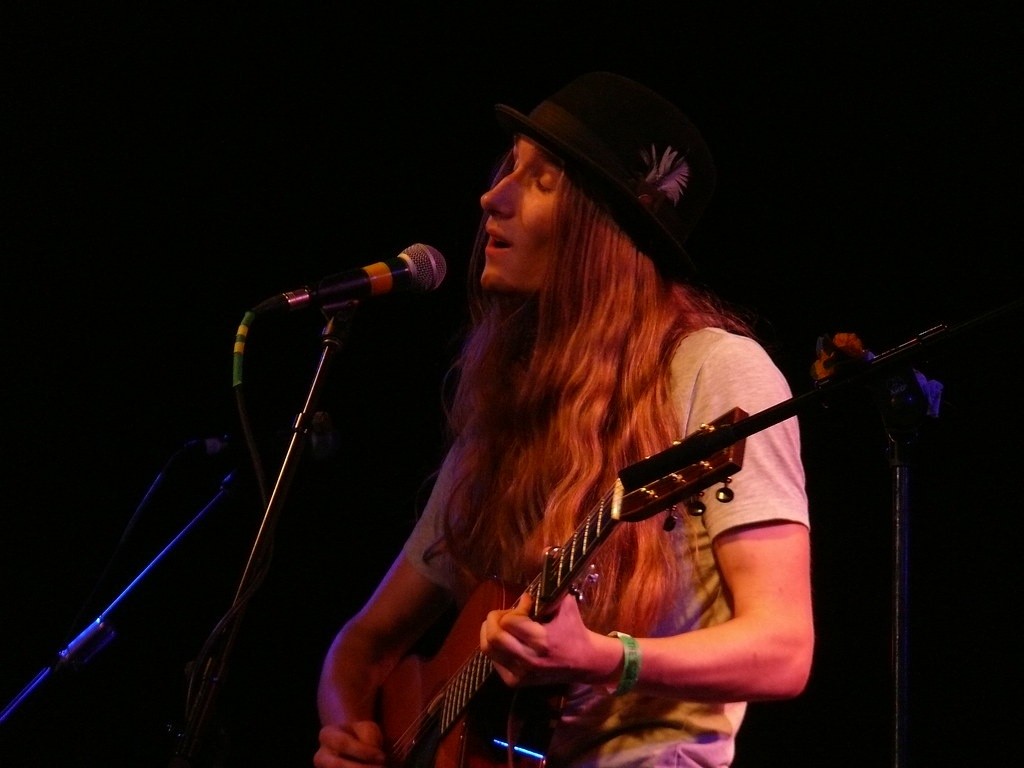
[[374, 407, 747, 768]]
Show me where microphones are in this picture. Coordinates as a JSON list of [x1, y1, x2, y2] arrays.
[[255, 243, 445, 316], [187, 410, 334, 454]]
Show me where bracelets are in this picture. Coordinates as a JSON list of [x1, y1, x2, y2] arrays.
[[605, 631, 641, 695]]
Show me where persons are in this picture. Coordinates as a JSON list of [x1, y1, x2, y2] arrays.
[[312, 58, 816, 768]]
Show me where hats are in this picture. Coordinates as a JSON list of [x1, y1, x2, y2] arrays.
[[497, 69, 716, 269]]
